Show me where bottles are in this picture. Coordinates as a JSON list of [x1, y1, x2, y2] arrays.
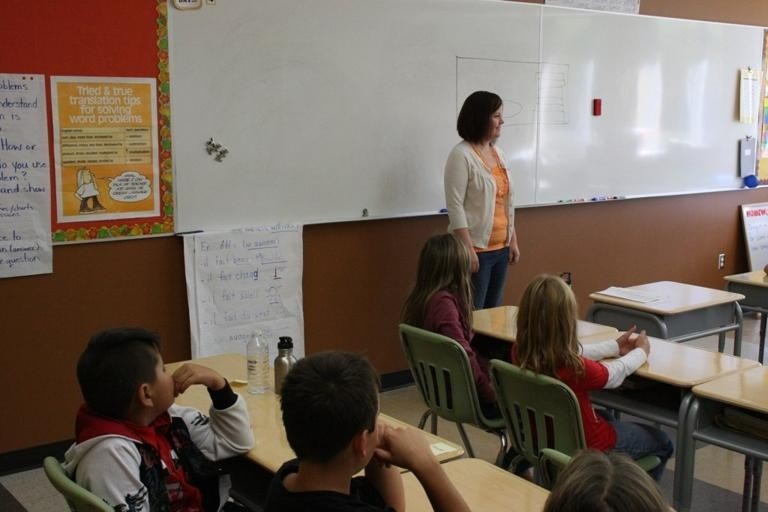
[[274, 336, 298, 394], [246, 327, 271, 395]]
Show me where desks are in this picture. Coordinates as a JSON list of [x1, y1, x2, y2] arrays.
[[723, 266, 768, 366], [563, 328, 762, 510], [584, 278, 746, 361], [454, 300, 621, 360], [159, 349, 466, 494], [387, 456, 554, 512], [676, 362, 767, 511]]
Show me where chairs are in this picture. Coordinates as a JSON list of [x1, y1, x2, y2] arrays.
[[396, 322, 507, 471], [41, 454, 115, 512], [486, 357, 662, 501]]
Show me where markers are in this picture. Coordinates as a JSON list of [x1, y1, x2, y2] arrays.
[[559, 195, 625, 202], [177, 230, 203, 234]]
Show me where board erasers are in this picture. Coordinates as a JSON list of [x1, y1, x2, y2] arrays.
[[594, 99, 601, 115], [441, 209, 448, 213]]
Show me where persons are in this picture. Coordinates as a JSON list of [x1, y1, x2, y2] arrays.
[[399, 234, 538, 476], [543, 448, 673, 511], [59, 327, 255, 512], [444, 91, 520, 310], [263, 350, 472, 512], [512, 274, 675, 483]]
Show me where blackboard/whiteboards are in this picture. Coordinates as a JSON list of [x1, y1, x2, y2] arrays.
[[741, 202, 768, 272], [167, 0, 765, 236]]
